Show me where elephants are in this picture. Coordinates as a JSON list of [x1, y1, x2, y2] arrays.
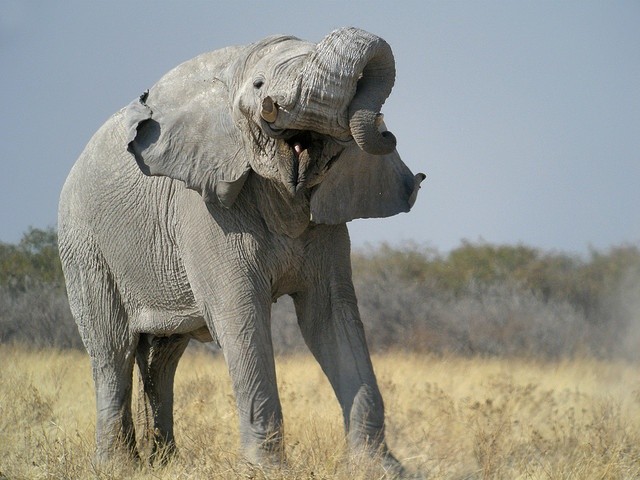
[[56, 26, 426, 480]]
